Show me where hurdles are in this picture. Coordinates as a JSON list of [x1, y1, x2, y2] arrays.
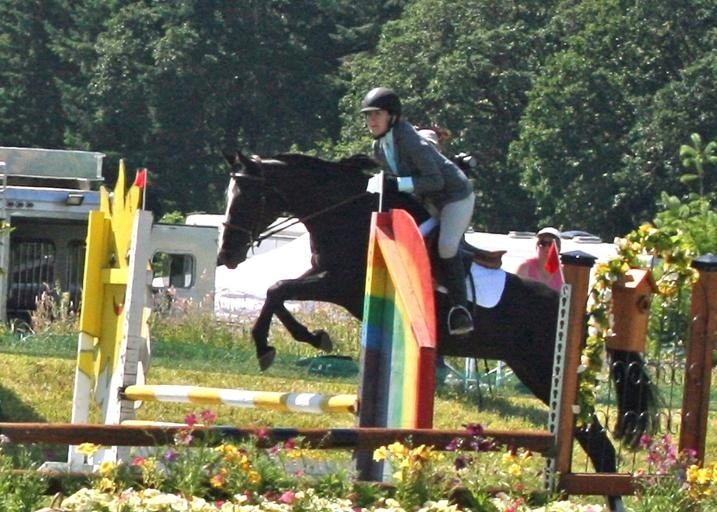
[[35, 208, 438, 484]]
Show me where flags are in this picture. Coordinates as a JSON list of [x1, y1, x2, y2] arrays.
[[543, 241, 559, 274], [133, 168, 144, 188], [364, 171, 384, 197]]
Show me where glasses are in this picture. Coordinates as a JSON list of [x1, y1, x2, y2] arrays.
[[537, 240, 552, 249]]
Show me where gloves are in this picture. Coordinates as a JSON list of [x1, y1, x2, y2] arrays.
[[383, 173, 398, 193]]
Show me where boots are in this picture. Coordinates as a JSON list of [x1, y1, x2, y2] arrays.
[[440, 255, 470, 329]]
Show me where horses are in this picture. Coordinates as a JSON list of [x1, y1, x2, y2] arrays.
[[216, 150, 668, 512]]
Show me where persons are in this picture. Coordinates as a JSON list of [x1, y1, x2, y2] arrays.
[[358, 85, 478, 335], [515, 225, 567, 291]]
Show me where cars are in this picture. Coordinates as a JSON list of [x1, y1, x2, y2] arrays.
[[9, 256, 53, 309]]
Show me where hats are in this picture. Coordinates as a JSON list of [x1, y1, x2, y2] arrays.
[[537, 228, 561, 254]]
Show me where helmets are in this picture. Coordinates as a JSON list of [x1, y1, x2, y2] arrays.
[[360, 87, 401, 116]]
